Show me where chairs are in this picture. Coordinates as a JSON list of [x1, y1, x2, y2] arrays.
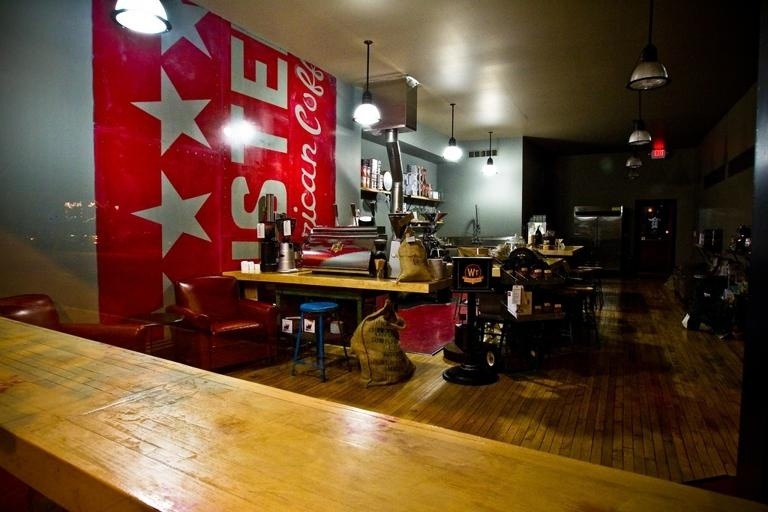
[[0, 294, 144, 354], [165, 275, 279, 371]]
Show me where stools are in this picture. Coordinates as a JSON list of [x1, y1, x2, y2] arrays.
[[568, 285, 597, 342], [290, 302, 351, 382], [572, 264, 604, 311]]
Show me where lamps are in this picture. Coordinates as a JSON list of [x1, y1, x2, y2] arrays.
[[351, 40, 381, 126], [624, 156, 643, 180], [111, 0, 172, 35], [627, 89, 651, 146], [442, 103, 462, 161], [624, 0, 670, 89], [482, 132, 496, 177]]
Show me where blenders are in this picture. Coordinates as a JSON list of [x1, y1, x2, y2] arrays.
[[277, 218, 299, 274]]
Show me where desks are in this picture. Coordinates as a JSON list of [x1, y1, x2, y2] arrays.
[[222, 246, 586, 354]]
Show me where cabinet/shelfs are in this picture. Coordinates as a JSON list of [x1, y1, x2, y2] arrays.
[[500, 247, 565, 372]]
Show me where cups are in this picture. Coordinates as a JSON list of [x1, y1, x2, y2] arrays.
[[427, 259, 443, 281], [241, 261, 261, 272]]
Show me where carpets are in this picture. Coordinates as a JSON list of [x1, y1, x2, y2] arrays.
[[309, 290, 504, 357]]
